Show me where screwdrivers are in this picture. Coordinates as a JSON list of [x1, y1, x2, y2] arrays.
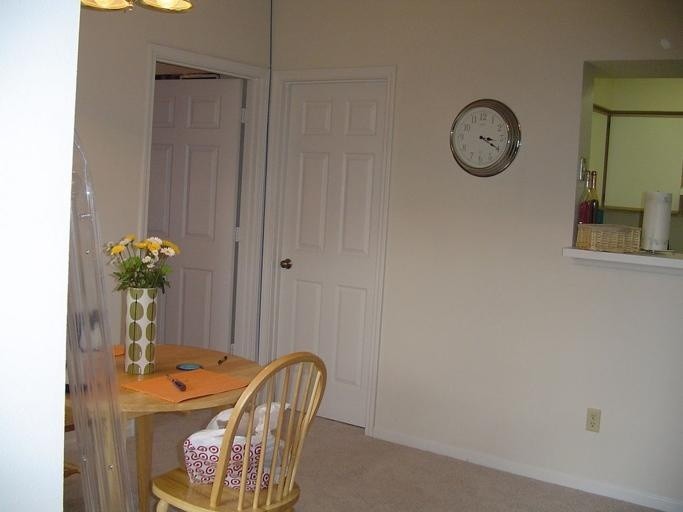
[[167, 374, 186, 391]]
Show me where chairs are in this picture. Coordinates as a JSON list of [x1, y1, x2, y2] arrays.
[[151, 352, 327, 512]]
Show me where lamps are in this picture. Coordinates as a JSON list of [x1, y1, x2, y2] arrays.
[[81, 0, 195, 15]]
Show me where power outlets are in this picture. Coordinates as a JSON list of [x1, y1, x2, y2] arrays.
[[585, 408, 600, 432]]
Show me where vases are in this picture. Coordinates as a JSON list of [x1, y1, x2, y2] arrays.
[[125, 287, 158, 374]]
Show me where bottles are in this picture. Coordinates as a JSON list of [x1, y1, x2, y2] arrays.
[[578, 170, 599, 224], [679, 176, 683, 212]]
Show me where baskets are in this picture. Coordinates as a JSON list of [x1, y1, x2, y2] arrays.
[[575, 221, 643, 254]]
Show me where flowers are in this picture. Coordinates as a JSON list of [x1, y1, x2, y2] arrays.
[[100, 233, 180, 294]]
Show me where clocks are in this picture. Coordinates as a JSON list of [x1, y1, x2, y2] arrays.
[[448, 98, 521, 177]]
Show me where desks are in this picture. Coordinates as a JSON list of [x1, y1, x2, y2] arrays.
[[66, 342, 265, 512]]
